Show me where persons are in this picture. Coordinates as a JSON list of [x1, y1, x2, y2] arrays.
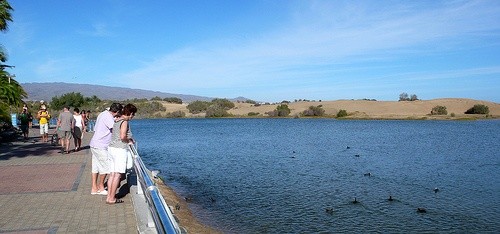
[[38, 100, 48, 116], [89, 103, 123, 195], [79, 109, 96, 133], [18, 109, 32, 141], [22, 103, 28, 114], [36, 105, 52, 143], [71, 108, 85, 152], [55, 105, 74, 154], [104, 103, 137, 204]]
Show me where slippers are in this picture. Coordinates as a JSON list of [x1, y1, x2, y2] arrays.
[[109, 198, 124, 205], [105, 199, 109, 204], [97, 190, 107, 195], [91, 190, 97, 194]]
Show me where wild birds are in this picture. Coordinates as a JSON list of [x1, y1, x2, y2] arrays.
[[293, 151, 296, 153], [433, 188, 439, 193], [364, 172, 371, 176], [325, 207, 335, 215], [292, 157, 295, 158], [416, 207, 426, 214], [388, 196, 393, 201], [347, 146, 351, 149], [355, 154, 360, 157], [351, 196, 360, 204]]
